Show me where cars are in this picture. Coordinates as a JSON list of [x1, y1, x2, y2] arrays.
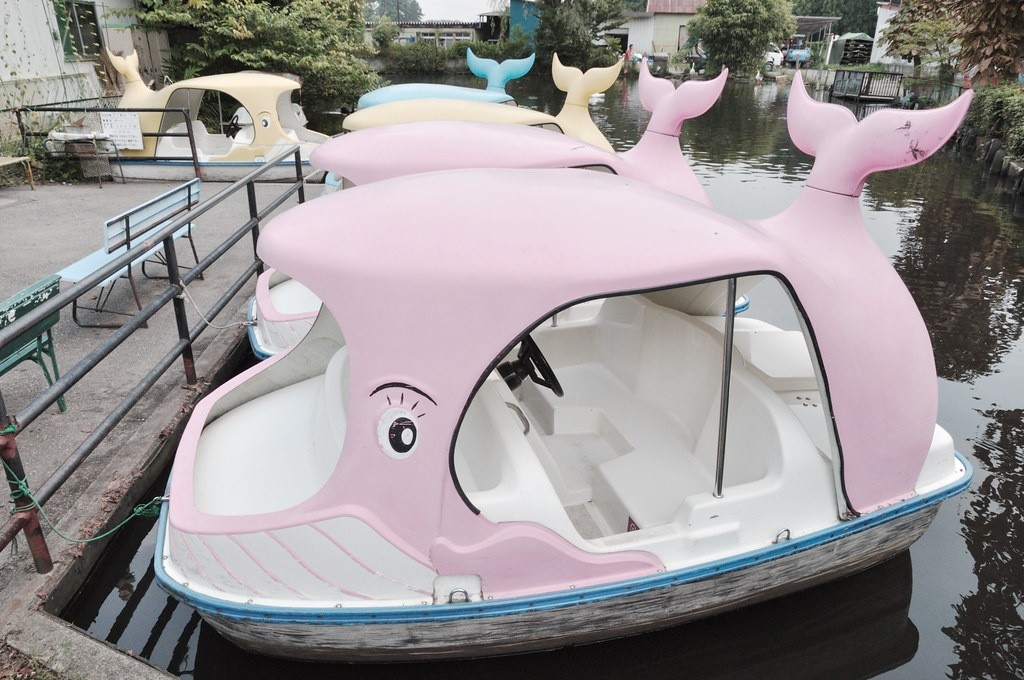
[[762, 42, 784, 71]]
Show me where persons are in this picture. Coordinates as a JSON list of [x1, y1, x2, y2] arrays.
[[623, 44, 634, 74]]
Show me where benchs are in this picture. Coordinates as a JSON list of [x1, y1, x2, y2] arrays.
[[524, 292, 841, 531], [0, 273, 66, 419], [56, 176, 202, 328], [157, 120, 232, 161]]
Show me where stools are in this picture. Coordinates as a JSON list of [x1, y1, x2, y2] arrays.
[[0, 157, 35, 190]]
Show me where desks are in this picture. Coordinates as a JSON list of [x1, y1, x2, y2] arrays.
[[41, 133, 125, 188]]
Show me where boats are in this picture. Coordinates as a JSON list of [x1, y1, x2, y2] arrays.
[[246, 58, 731, 361], [308, 45, 627, 208], [153, 60, 978, 667], [101, 46, 336, 184]]
